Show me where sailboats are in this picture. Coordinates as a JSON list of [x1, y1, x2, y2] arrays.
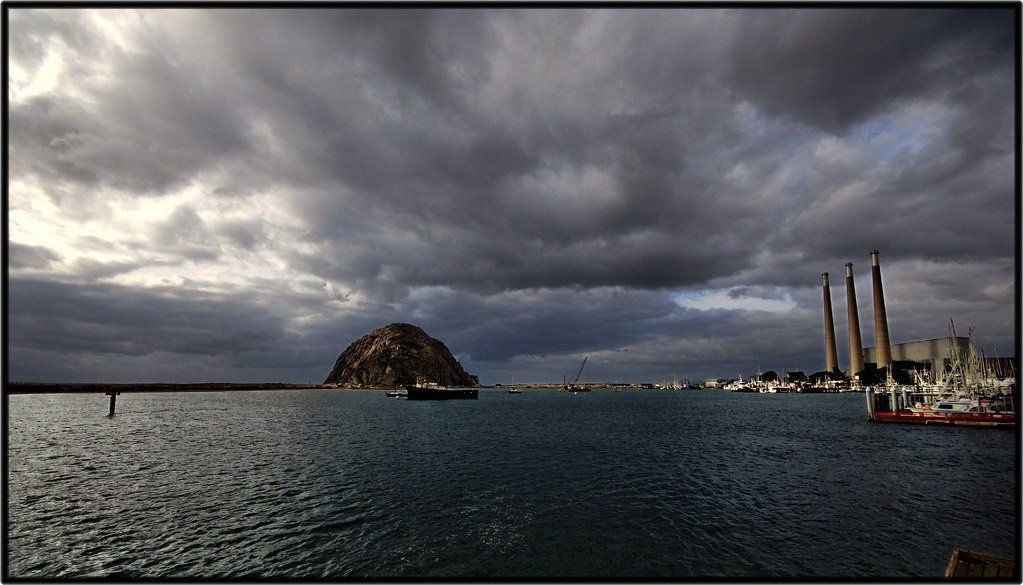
[[563, 355, 592, 392]]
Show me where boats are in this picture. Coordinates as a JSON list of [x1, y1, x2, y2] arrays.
[[723, 374, 866, 394], [385, 392, 408, 397], [653, 382, 694, 391], [508, 387, 522, 394], [400, 375, 480, 401], [865, 315, 1016, 428]]
